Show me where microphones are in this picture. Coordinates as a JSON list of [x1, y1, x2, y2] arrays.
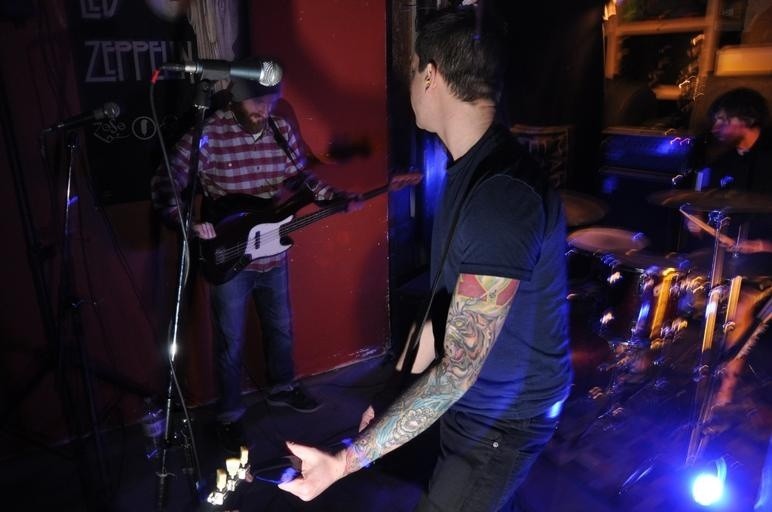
[[161, 59, 283, 88], [49, 102, 120, 132]]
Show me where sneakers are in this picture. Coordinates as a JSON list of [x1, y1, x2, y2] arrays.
[[216, 422, 253, 455], [268, 390, 324, 415]]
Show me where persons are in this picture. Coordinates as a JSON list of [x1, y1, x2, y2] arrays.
[[276, 10, 575, 512], [618, 86, 772, 434], [150, 55, 366, 457]]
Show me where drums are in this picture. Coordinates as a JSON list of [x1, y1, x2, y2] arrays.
[[609, 255, 685, 354], [565, 228, 651, 337]]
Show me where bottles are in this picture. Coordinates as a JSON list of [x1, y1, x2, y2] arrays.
[[139, 397, 168, 464]]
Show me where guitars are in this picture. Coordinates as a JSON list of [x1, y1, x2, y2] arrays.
[[184, 168, 425, 285], [205, 379, 438, 512]]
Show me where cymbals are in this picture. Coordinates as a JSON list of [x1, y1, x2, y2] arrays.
[[648, 186, 772, 216]]
